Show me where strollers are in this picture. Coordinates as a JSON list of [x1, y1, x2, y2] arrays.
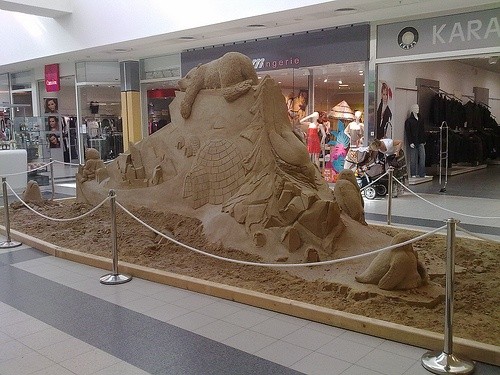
[[356, 154, 400, 200]]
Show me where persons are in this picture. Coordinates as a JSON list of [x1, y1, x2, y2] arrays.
[[45, 99, 58, 113], [344, 111, 365, 170], [300, 112, 327, 165], [48, 116, 59, 131], [283, 89, 307, 122], [404, 104, 428, 179], [49, 133, 60, 148], [367, 138, 409, 198], [377, 83, 392, 138], [4, 119, 15, 143]]
